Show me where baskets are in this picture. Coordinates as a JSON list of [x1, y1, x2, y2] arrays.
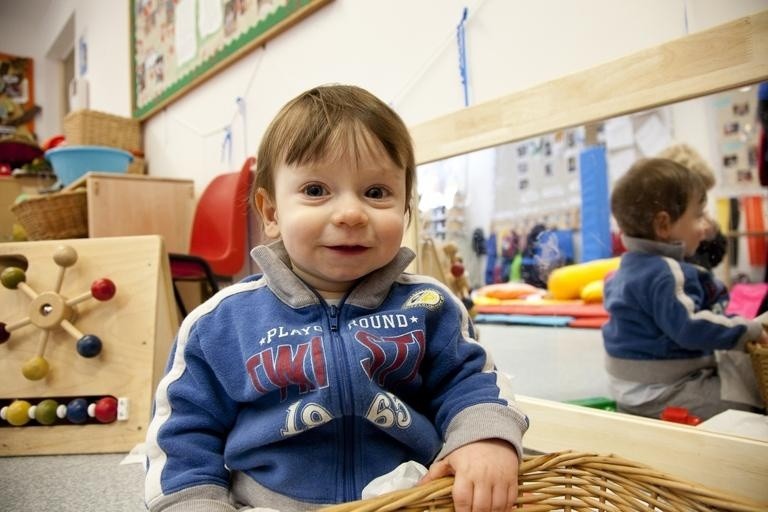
[[8, 187, 93, 240], [310, 447, 767, 511], [127, 158, 147, 174], [61, 108, 143, 154]]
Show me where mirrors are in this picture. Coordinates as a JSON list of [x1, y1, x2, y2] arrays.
[[403, 8, 768, 503]]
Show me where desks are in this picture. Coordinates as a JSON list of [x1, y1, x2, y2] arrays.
[[60, 172, 196, 253]]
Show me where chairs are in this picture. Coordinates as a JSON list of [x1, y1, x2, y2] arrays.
[[168, 157, 256, 319]]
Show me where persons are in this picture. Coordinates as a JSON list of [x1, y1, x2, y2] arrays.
[[660, 140, 729, 274], [141, 81, 531, 510], [600, 153, 768, 423]]
